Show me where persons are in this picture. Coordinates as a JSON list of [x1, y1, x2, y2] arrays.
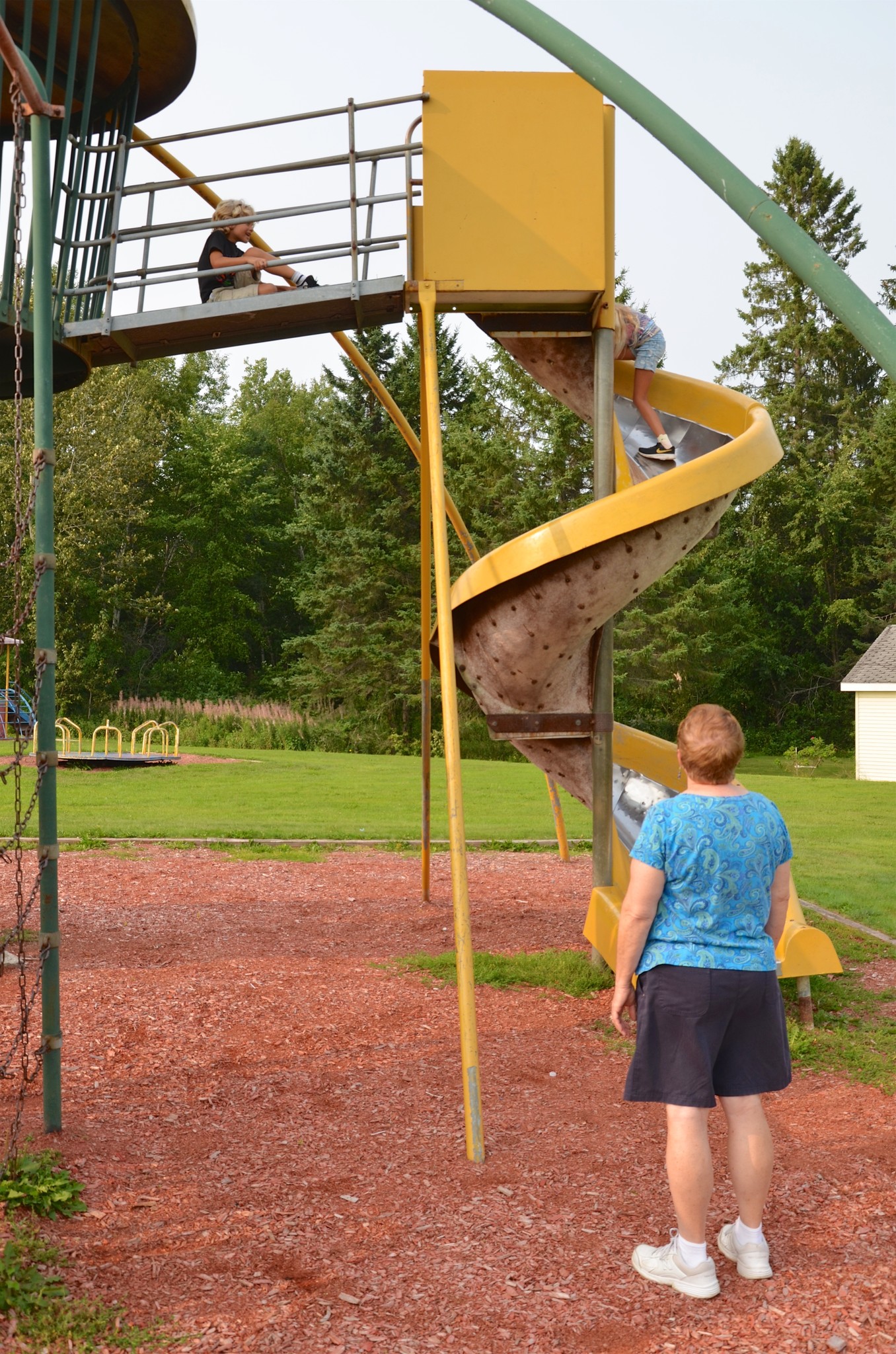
[[608, 703, 793, 1300], [614, 302, 675, 460], [197, 199, 321, 304]]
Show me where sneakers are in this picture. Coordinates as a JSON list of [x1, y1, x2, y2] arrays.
[[632, 1225, 721, 1298], [716, 1218, 773, 1280]]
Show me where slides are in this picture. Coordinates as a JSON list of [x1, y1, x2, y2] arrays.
[[424, 311, 851, 985]]
